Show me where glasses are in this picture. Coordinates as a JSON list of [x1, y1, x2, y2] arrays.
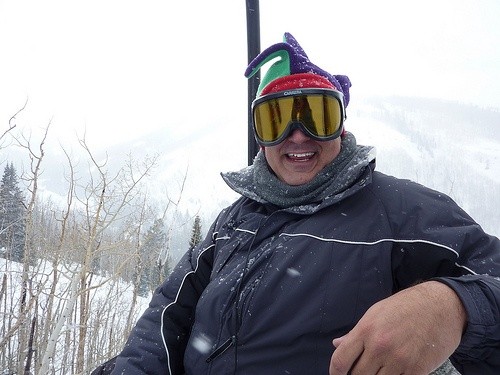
[[250, 88, 346, 147]]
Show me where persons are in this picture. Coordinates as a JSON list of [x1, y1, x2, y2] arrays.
[[88, 32, 500, 375]]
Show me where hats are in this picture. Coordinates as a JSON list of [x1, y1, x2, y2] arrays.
[[244, 30, 352, 109]]
